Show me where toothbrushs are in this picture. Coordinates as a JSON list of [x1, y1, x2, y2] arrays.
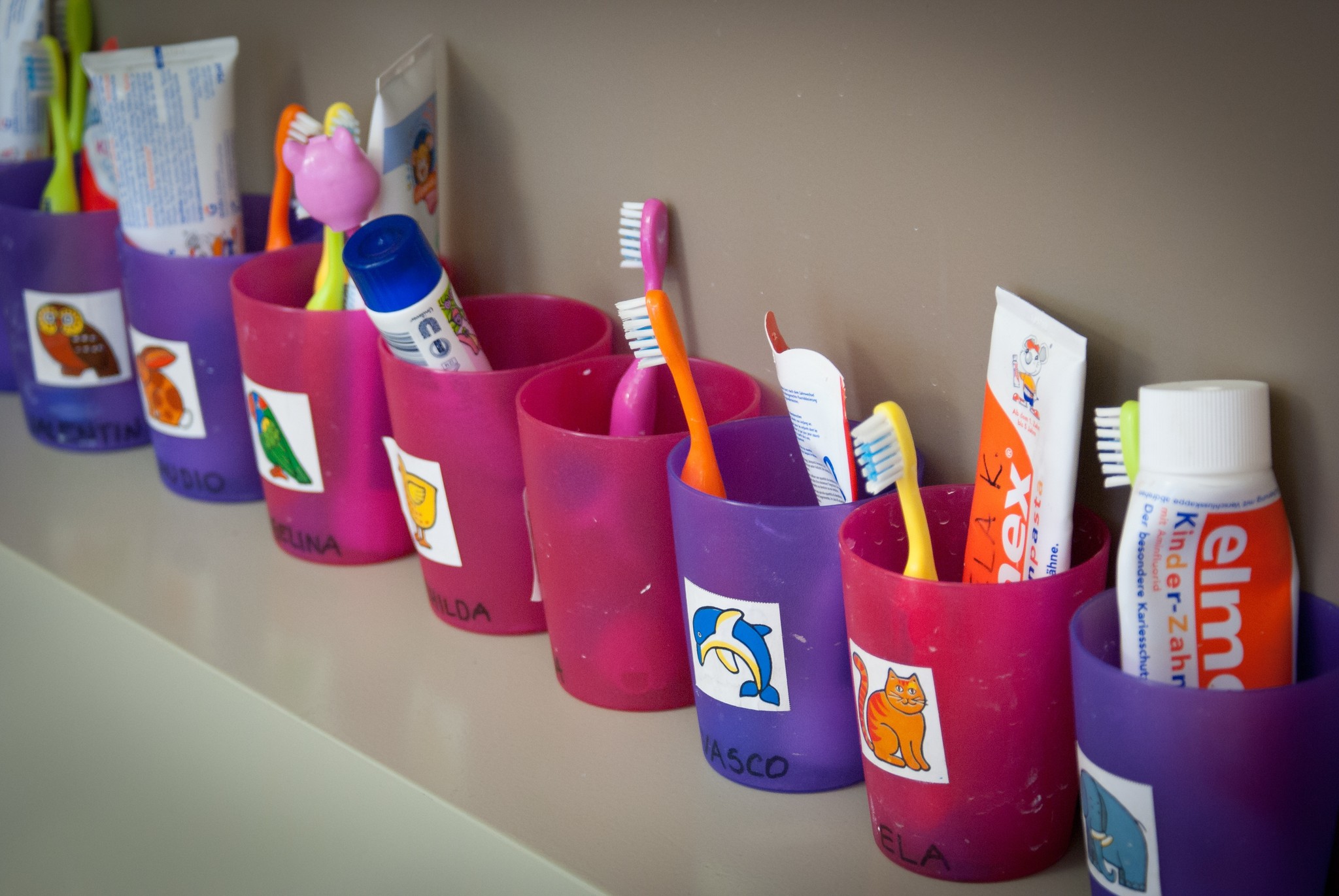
[[615, 290, 731, 503], [848, 400, 948, 584], [1087, 404, 1145, 492], [604, 198, 675, 440], [20, 0, 96, 212], [264, 99, 381, 316]]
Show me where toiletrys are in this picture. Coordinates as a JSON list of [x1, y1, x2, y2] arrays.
[[0, 0, 51, 166], [755, 308, 863, 505], [959, 284, 1088, 581], [339, 213, 498, 372], [79, 34, 247, 255], [346, 31, 455, 314], [1116, 379, 1299, 692]]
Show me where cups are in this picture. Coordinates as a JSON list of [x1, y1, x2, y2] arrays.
[[0, 141, 1339, 896]]
